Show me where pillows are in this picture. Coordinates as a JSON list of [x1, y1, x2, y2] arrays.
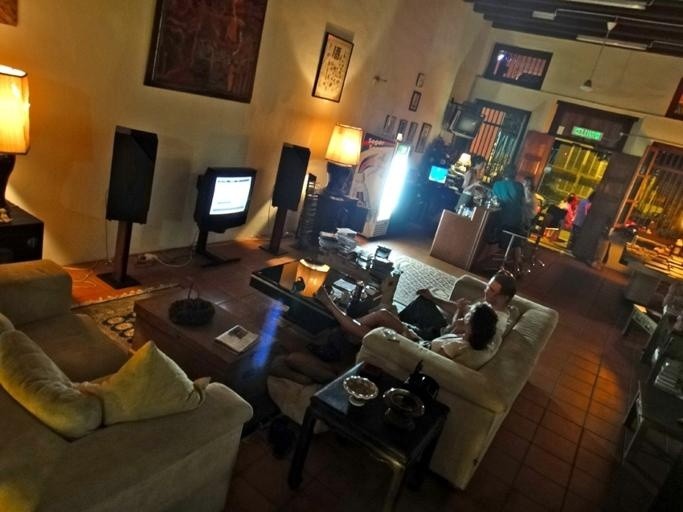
[[1, 328, 103, 440], [0, 312, 16, 334], [64, 339, 213, 428]]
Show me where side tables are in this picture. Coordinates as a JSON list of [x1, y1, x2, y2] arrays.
[[286, 359, 452, 512], [618, 378, 683, 465], [620, 302, 664, 353]]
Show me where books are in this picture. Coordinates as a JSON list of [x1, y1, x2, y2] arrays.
[[215, 325, 262, 353], [655, 358, 683, 398]]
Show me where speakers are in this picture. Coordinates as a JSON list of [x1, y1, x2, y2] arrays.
[[273, 143, 311, 212], [104, 125, 160, 226]]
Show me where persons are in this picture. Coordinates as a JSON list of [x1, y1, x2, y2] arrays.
[[492, 164, 527, 271], [518, 177, 537, 227], [569, 193, 595, 247], [458, 155, 486, 212], [309, 285, 498, 366], [402, 270, 517, 340]]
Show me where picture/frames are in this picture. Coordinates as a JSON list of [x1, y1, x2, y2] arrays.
[[395, 119, 408, 139], [408, 89, 422, 112], [416, 74, 426, 89], [415, 122, 433, 154], [404, 121, 419, 144], [143, 0, 270, 103], [310, 31, 355, 105], [381, 113, 398, 135]]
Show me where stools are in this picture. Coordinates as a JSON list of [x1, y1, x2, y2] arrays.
[[524, 225, 546, 274], [483, 225, 529, 282]]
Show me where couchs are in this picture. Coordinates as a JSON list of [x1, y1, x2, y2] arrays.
[[0, 258, 254, 512], [354, 273, 561, 492]]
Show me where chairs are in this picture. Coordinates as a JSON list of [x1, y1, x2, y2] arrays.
[[648, 337, 683, 397], [542, 204, 568, 243]]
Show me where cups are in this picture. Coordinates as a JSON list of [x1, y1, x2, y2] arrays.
[[324, 280, 334, 295], [341, 291, 353, 305]]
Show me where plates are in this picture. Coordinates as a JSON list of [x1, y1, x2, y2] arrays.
[[343, 375, 379, 407], [168, 298, 215, 328]]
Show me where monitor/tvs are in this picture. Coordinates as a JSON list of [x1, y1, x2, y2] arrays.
[[191, 166, 258, 234], [442, 106, 485, 141], [425, 163, 449, 183]]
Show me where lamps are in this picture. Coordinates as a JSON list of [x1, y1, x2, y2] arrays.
[[580, 16, 620, 91], [0, 62, 33, 223], [322, 122, 363, 200]]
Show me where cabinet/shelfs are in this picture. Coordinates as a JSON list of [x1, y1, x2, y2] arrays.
[[0, 198, 45, 266], [309, 186, 359, 248], [429, 203, 504, 272], [619, 235, 683, 305]]
